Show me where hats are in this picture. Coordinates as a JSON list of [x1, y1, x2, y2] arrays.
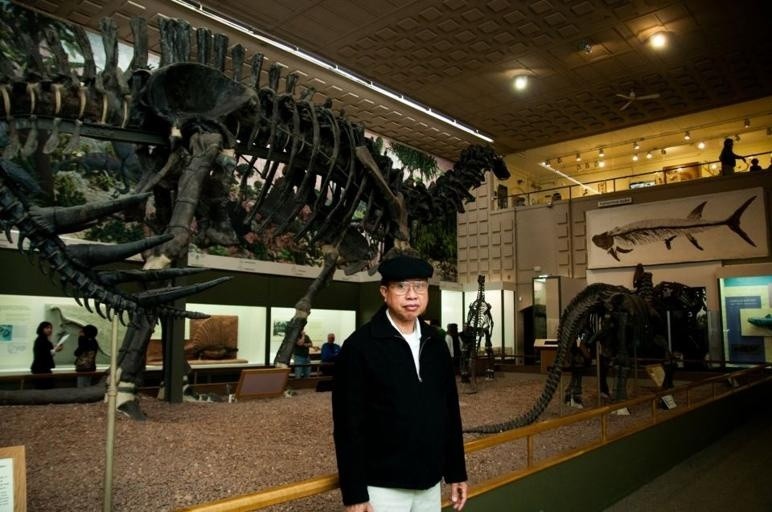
[[378, 255, 434, 281]]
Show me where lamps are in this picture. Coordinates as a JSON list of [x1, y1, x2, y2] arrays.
[[545, 122, 772, 177]]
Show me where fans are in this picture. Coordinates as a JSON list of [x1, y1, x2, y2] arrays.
[[617, 89, 659, 113]]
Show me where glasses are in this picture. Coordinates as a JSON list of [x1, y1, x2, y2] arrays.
[[391, 281, 429, 290]]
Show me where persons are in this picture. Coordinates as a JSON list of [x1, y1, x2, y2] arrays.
[[31, 320, 64, 375], [330, 253, 470, 510], [511, 177, 525, 206], [320, 332, 340, 361], [294, 329, 313, 378], [749, 157, 762, 172], [717, 138, 745, 176], [73, 324, 98, 387]]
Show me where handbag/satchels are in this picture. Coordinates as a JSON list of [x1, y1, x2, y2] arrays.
[[74, 351, 97, 372]]
